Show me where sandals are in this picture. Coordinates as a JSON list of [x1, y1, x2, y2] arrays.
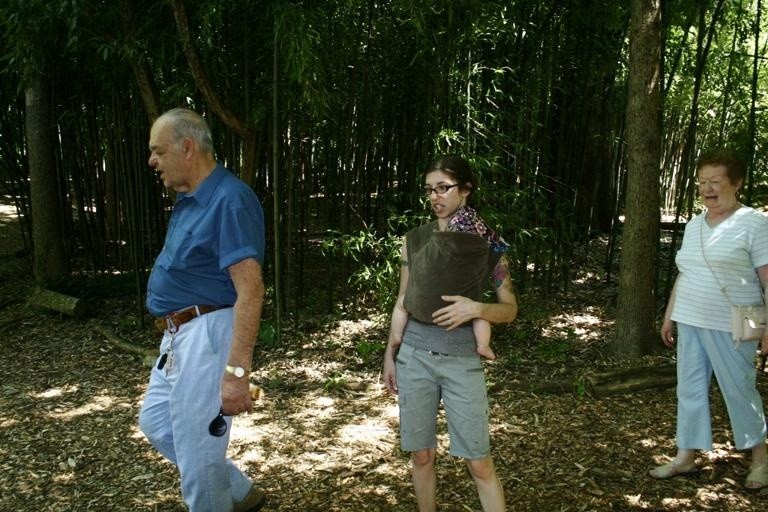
[[649, 458, 698, 479], [744, 462, 768, 491]]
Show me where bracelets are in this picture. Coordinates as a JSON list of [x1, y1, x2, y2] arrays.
[[663, 315, 673, 321]]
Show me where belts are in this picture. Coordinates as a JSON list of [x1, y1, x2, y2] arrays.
[[155, 302, 233, 332]]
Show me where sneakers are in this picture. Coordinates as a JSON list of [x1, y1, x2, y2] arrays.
[[234, 487, 266, 512]]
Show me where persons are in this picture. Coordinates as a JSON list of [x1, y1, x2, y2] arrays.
[[648, 149, 767, 491], [384, 153, 518, 511], [136, 106, 268, 511], [399, 206, 496, 361]]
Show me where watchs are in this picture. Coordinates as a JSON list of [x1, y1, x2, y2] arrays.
[[224, 363, 252, 378]]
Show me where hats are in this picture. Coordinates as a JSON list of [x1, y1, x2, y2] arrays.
[[447, 209, 487, 235]]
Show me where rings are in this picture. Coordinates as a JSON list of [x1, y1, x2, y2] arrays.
[[448, 319, 454, 323]]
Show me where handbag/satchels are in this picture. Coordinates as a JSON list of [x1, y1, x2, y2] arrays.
[[731, 304, 768, 341]]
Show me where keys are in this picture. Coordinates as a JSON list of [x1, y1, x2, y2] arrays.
[[157, 332, 176, 370]]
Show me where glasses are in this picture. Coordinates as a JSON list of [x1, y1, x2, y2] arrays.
[[423, 182, 460, 194], [209, 406, 234, 436]]
